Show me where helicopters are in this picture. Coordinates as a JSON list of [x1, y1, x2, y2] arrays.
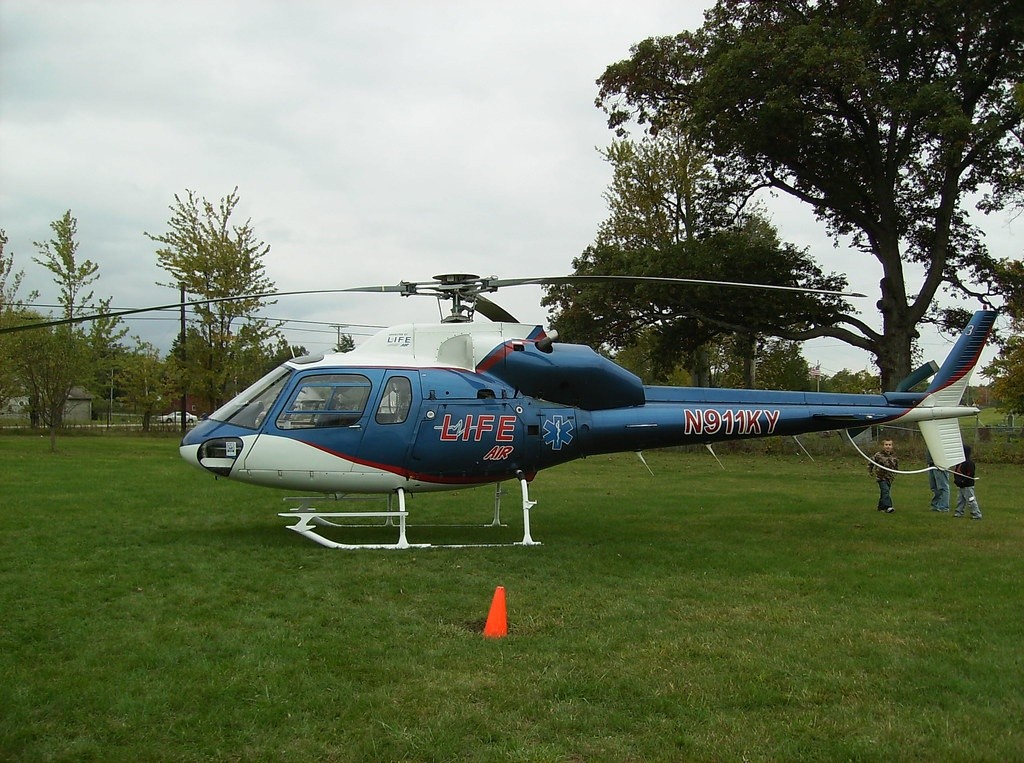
[[0, 270, 1000, 550]]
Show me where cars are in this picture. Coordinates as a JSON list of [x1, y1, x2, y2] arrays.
[[156, 411, 199, 423]]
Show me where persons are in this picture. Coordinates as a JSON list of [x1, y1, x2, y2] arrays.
[[867, 437, 900, 512], [952, 444, 982, 519], [926, 448, 949, 512]]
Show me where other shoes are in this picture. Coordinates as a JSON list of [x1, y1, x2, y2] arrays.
[[937, 508, 949, 512], [880, 509, 886, 512], [886, 507, 894, 513], [971, 514, 982, 519], [953, 512, 964, 517], [930, 506, 938, 511]]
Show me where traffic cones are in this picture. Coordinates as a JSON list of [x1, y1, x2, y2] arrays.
[[480, 585, 509, 641]]
